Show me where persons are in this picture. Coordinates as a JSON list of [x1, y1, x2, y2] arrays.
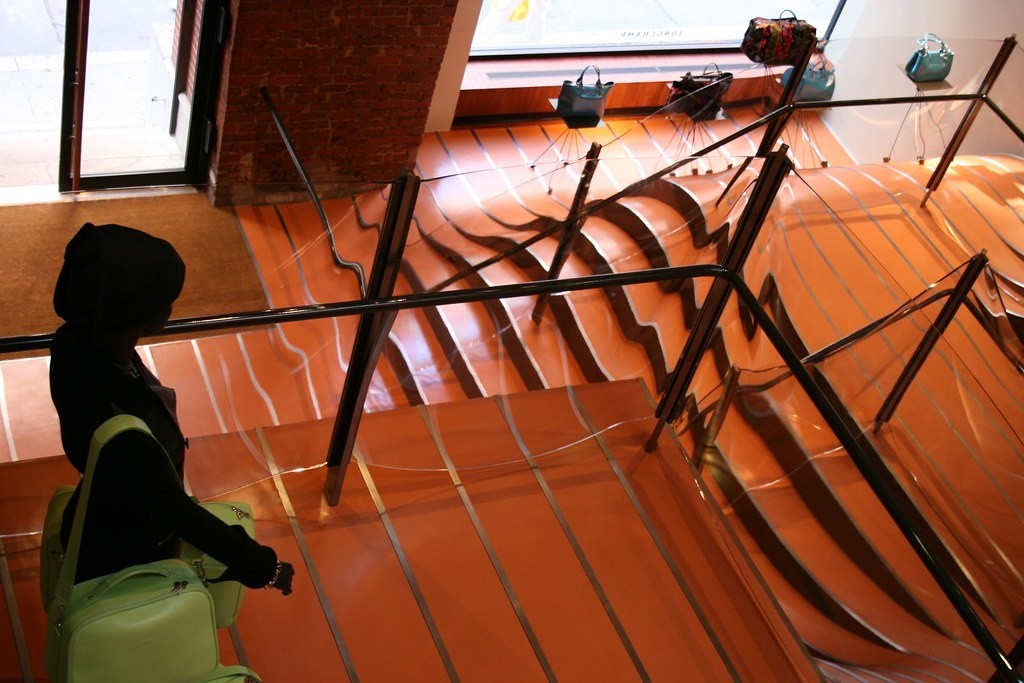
[[55, 220, 294, 615]]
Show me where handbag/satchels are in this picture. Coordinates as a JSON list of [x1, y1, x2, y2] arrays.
[[665, 62, 733, 120], [557, 65, 615, 127], [781, 61, 835, 100], [43, 414, 221, 682], [905, 33, 953, 83], [40, 484, 255, 630], [740, 10, 816, 64]]
